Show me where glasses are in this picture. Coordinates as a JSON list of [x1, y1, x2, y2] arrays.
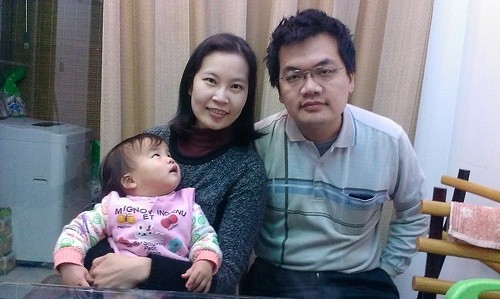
[[278, 64, 345, 86]]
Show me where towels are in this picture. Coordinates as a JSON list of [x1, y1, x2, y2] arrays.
[[448, 201, 497, 249]]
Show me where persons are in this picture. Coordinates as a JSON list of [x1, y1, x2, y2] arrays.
[[239, 8, 432, 299], [53, 133, 223, 294], [84, 32, 266, 296]]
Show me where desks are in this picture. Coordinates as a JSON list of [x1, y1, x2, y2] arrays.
[[0, 280, 261, 299]]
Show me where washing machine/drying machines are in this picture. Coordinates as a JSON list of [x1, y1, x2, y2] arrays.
[[1, 113, 93, 263]]
